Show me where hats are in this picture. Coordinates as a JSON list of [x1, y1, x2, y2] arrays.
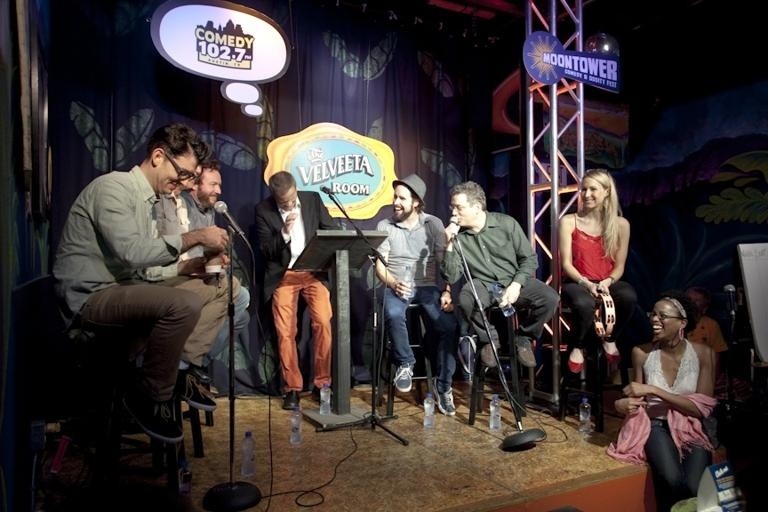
[[393, 174, 426, 206]]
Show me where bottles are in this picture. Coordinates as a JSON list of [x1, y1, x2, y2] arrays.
[[288, 410, 301, 443], [490, 284, 514, 319], [241, 431, 257, 474], [178, 460, 192, 499], [321, 383, 330, 416], [400, 265, 413, 302], [490, 395, 500, 430], [424, 392, 435, 429], [578, 396, 591, 434]]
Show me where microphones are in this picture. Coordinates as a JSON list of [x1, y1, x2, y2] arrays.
[[214, 201, 246, 238], [449, 216, 459, 243], [319, 184, 335, 194]]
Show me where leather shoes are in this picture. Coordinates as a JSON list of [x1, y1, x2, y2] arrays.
[[282, 390, 300, 410], [187, 362, 213, 385], [602, 341, 620, 364], [311, 385, 333, 402], [568, 347, 585, 373]]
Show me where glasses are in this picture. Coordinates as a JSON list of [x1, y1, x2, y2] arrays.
[[647, 312, 682, 320], [165, 151, 196, 181]]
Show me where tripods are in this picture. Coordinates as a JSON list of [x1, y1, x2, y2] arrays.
[[315, 194, 410, 446]]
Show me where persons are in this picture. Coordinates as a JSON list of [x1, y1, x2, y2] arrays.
[[615, 292, 719, 512], [556, 170, 638, 373], [141, 160, 205, 281], [53, 122, 229, 442], [682, 287, 730, 382], [180, 158, 251, 395], [440, 180, 561, 369], [252, 170, 339, 410], [375, 174, 461, 415]]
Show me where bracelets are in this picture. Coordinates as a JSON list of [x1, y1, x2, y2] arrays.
[[578, 276, 588, 285]]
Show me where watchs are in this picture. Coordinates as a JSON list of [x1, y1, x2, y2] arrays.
[[608, 276, 615, 284]]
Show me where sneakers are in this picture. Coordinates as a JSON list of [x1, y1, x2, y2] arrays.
[[514, 336, 536, 367], [479, 343, 499, 367], [121, 398, 184, 443], [432, 377, 456, 416], [393, 363, 414, 392], [174, 366, 217, 411]]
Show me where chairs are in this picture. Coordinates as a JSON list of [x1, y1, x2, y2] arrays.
[[171, 355, 214, 456], [559, 297, 631, 433], [12, 277, 187, 508], [361, 258, 435, 418], [467, 304, 527, 426]]
